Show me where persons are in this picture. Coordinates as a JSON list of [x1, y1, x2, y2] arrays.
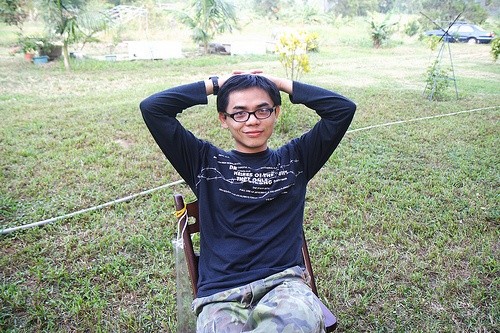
[[140, 69, 357, 333]]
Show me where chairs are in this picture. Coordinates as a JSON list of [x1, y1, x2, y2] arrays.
[[173, 195, 337, 333]]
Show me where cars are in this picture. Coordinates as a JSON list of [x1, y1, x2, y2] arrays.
[[425, 21, 494, 45]]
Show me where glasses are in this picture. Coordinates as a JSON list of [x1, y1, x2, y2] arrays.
[[223, 104, 276, 122]]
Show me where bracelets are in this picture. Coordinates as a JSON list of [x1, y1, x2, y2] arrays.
[[209, 76, 219, 95]]
